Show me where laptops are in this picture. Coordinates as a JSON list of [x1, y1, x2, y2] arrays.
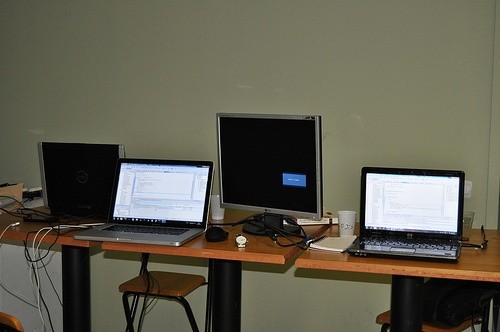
[[73, 158, 216, 247], [347, 167, 465, 263], [37, 142, 124, 220]]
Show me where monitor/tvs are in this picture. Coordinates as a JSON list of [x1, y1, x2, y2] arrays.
[[215, 112, 323, 236]]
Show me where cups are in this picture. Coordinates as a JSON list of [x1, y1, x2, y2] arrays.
[[462, 210, 476, 242], [210, 195, 225, 220], [337, 210, 357, 238]]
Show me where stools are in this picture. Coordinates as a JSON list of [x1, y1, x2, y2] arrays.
[[119, 271, 205, 332], [376, 310, 479, 332]]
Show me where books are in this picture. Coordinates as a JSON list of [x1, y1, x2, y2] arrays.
[[308, 235, 357, 252], [293, 211, 338, 225]]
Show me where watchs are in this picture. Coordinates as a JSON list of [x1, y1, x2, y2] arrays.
[[235, 233, 249, 248]]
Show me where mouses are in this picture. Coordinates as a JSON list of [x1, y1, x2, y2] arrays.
[[206, 227, 228, 241]]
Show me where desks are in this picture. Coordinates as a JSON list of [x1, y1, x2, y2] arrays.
[[296, 221, 500, 332], [0, 206, 100, 332], [102, 207, 329, 332]]
